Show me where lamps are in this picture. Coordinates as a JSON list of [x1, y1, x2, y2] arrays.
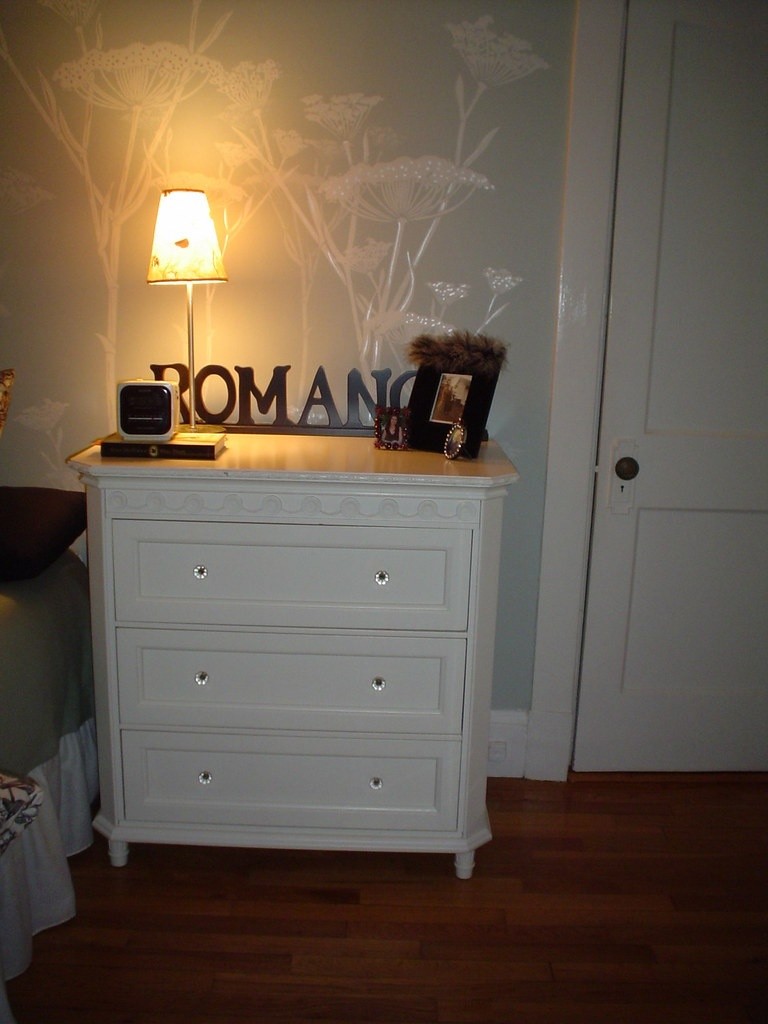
[[146, 188, 229, 434]]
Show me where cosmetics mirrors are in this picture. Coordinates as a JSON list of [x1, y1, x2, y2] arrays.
[[445, 426, 466, 460]]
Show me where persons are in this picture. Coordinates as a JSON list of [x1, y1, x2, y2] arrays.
[[434, 379, 457, 419], [382, 415, 402, 444]]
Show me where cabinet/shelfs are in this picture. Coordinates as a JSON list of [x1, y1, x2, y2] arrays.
[[66, 434, 520, 881]]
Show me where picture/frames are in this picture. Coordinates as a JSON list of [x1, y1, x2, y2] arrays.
[[405, 331, 508, 457], [374, 405, 412, 452], [445, 424, 473, 459]]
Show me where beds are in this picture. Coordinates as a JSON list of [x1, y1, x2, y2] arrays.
[[0, 547, 98, 1024]]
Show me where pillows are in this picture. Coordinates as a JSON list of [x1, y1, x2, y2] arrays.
[[0, 369, 14, 433], [0, 484, 86, 583]]
